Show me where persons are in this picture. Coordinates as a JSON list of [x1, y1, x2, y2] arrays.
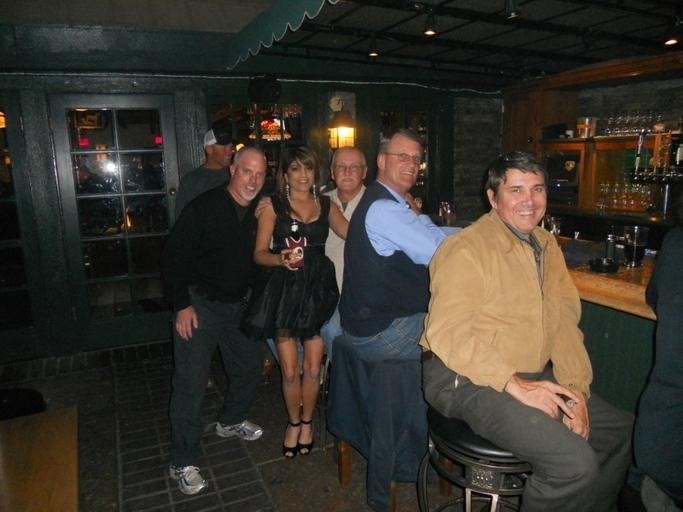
[[239, 143, 349, 459], [420, 151, 636, 510], [338, 128, 463, 364], [156, 147, 271, 496], [252, 145, 419, 385], [173, 126, 234, 226], [635, 221, 682, 510]]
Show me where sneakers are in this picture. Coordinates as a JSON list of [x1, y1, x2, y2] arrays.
[[169, 462, 208, 495], [216, 420, 264, 442]]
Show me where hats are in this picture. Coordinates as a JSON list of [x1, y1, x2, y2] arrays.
[[203, 128, 238, 150]]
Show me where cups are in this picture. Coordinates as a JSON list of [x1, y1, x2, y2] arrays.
[[620, 225, 647, 267], [541, 215, 561, 249]]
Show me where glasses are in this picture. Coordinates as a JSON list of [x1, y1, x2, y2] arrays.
[[380, 150, 422, 165]]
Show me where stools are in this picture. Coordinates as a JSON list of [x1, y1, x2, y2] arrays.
[[325, 333, 453, 511], [416, 407, 532, 511]]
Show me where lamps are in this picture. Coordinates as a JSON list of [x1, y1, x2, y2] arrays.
[[365, 38, 380, 57], [664, 21, 679, 47], [503, 0, 521, 21], [326, 95, 357, 151], [422, 12, 439, 35]]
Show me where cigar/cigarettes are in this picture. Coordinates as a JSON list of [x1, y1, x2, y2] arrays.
[[560, 393, 575, 409]]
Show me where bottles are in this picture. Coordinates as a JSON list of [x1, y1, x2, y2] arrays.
[[436, 200, 456, 219], [595, 181, 656, 213], [606, 225, 618, 236], [284, 218, 309, 269], [603, 109, 665, 135], [605, 234, 624, 266], [658, 135, 683, 171]]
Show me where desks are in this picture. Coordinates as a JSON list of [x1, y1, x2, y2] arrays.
[[547, 202, 677, 252], [0, 404, 80, 512]]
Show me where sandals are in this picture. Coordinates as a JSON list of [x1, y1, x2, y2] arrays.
[[282, 422, 301, 459], [298, 419, 314, 455]]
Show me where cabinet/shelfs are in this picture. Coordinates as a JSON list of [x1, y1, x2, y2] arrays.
[[528, 130, 683, 215]]
